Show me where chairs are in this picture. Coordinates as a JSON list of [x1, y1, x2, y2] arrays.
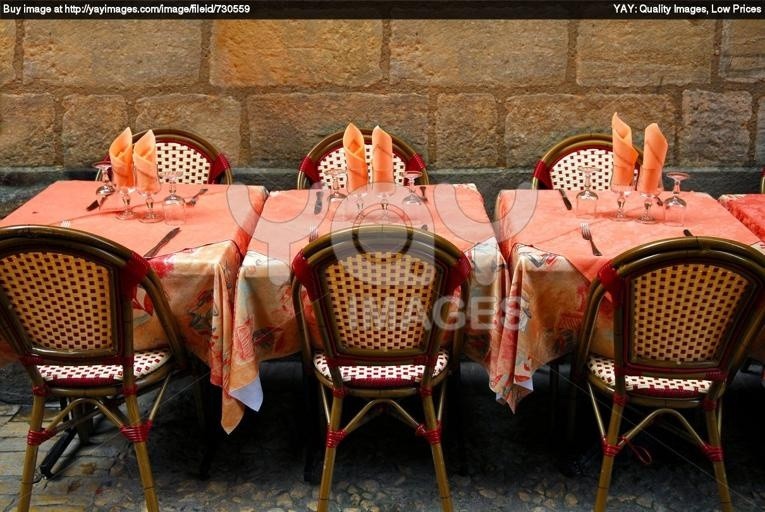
[[535, 132, 645, 190], [296, 128, 432, 189], [95, 126, 233, 182], [289, 221, 473, 511], [1, 225, 196, 511], [573, 235, 758, 512]]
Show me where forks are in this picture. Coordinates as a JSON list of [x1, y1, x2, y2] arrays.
[[185, 189, 208, 208], [308, 223, 318, 244], [581, 223, 602, 257]]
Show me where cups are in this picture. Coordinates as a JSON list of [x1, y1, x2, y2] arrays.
[[111, 162, 137, 220], [610, 165, 636, 221], [637, 165, 663, 225], [662, 172, 691, 228], [369, 168, 397, 226], [346, 167, 369, 226], [135, 163, 161, 223], [400, 171, 425, 228], [94, 162, 118, 216], [322, 169, 354, 223], [575, 167, 599, 222], [162, 171, 187, 227]]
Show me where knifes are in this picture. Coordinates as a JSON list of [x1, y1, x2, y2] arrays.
[[313, 190, 324, 215], [143, 227, 181, 260], [558, 188, 572, 211]]
